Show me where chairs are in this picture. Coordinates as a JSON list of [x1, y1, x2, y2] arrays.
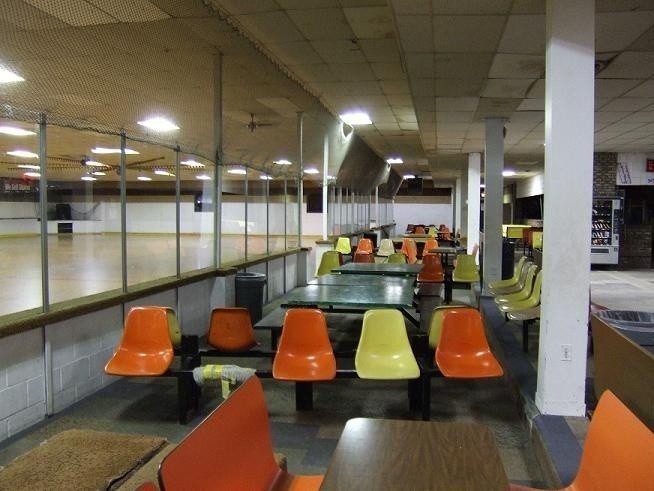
[[487, 259, 543, 354], [282, 236, 481, 326], [405, 225, 453, 245], [503, 225, 542, 259], [104, 305, 503, 424]]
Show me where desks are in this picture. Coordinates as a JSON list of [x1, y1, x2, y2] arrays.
[[319, 418, 511, 490]]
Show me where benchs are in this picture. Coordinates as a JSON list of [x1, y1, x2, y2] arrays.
[[509, 390, 652, 491], [590, 315, 652, 431], [134, 480, 158, 491], [157, 374, 325, 490]]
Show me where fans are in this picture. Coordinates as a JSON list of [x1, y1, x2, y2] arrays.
[[233, 112, 281, 134]]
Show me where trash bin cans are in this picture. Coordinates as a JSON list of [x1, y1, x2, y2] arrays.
[[364, 232, 377, 248], [235, 272, 267, 326], [502, 237, 515, 280]]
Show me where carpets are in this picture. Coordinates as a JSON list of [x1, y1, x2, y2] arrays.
[[0, 427, 287, 491]]
